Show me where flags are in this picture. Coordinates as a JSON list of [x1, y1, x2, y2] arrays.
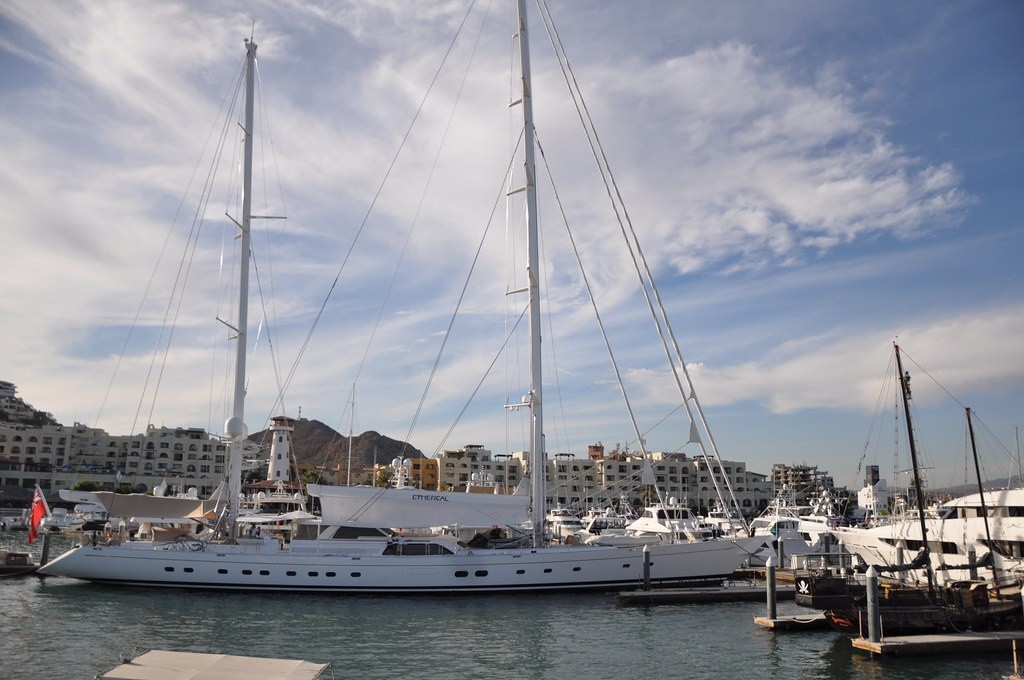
[[28, 487, 48, 544]]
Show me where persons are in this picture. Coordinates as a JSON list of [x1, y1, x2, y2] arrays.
[[249, 524, 260, 537], [396, 536, 405, 555]]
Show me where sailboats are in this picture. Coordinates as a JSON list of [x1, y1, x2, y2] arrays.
[[34, 1, 779, 589], [545, 341, 1024, 636]]
